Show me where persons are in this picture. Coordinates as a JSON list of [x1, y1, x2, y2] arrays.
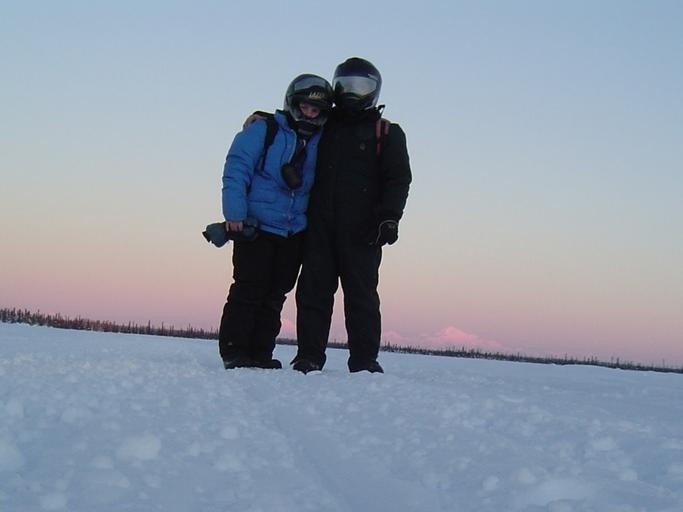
[[218, 73, 334, 370], [242, 57, 413, 374]]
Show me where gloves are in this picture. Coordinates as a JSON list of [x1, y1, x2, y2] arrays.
[[202, 218, 260, 248], [374, 214, 402, 247]]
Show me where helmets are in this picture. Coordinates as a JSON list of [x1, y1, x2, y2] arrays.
[[283, 73, 334, 138], [331, 56, 382, 111]]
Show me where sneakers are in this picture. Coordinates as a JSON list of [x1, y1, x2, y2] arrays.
[[290, 354, 327, 376], [348, 356, 384, 373], [223, 356, 281, 369]]
